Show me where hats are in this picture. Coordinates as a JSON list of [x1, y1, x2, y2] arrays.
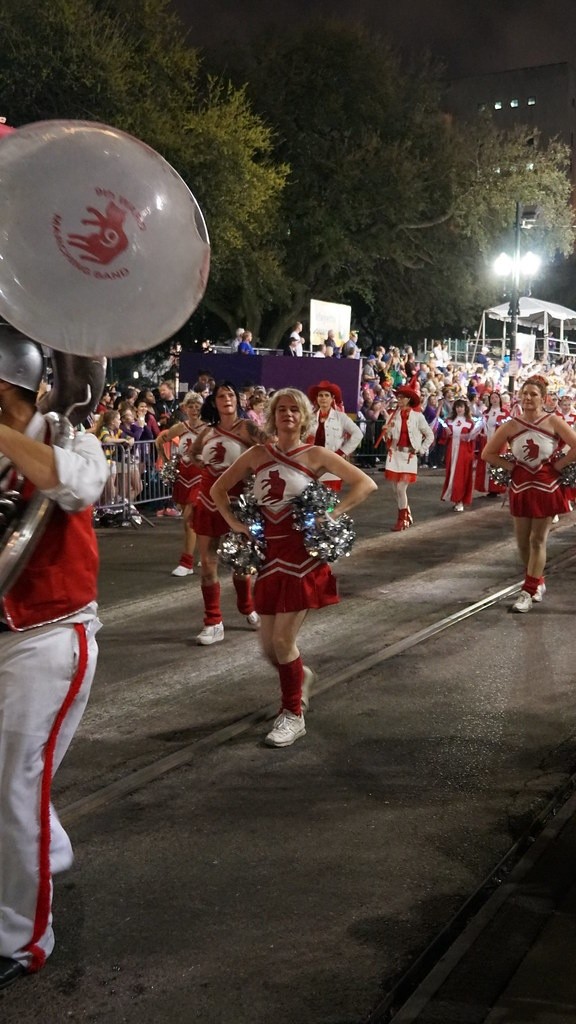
[[307, 380, 342, 405], [391, 386, 421, 408], [368, 355, 377, 360]]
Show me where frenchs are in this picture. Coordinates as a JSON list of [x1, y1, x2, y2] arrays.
[[0, 118, 212, 602]]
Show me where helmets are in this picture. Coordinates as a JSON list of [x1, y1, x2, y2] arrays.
[[0, 322, 45, 392]]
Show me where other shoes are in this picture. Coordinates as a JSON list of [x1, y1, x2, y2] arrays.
[[550, 514, 559, 523], [164, 508, 182, 516], [453, 503, 464, 512], [156, 510, 164, 517]]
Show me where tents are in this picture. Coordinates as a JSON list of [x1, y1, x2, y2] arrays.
[[482, 295, 576, 361]]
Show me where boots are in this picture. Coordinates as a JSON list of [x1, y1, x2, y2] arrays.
[[391, 506, 413, 531]]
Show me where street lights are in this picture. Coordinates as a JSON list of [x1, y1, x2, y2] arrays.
[[492, 199, 541, 408]]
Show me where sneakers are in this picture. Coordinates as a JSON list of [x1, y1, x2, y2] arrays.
[[246, 611, 262, 629], [532, 583, 547, 602], [172, 566, 193, 577], [300, 666, 314, 713], [513, 591, 533, 612], [195, 622, 225, 644], [265, 708, 306, 747]]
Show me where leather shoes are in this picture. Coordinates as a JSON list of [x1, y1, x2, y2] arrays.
[[0, 956, 24, 988]]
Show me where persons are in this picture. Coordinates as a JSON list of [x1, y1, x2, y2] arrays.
[[0, 320, 576, 576], [0, 334, 106, 979], [489, 379, 574, 615], [189, 382, 270, 646], [211, 387, 379, 746]]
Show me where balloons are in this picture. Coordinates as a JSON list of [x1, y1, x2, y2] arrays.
[[41, 342, 106, 426], [0, 119, 211, 359]]
